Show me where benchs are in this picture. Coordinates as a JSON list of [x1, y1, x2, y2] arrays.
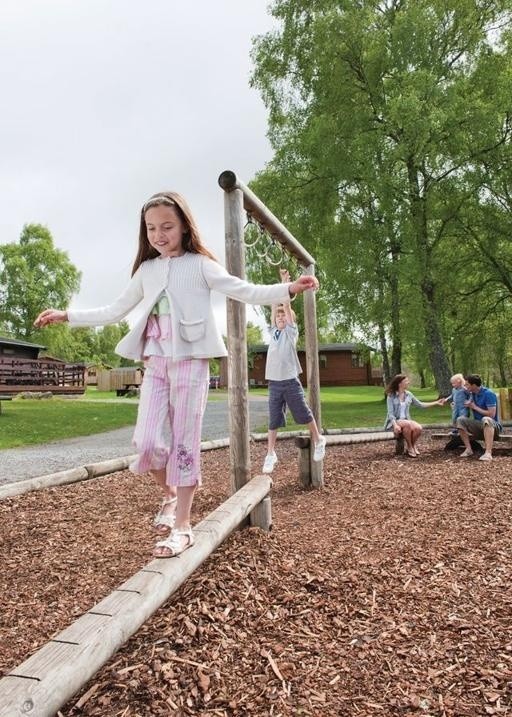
[[435, 432, 510, 452]]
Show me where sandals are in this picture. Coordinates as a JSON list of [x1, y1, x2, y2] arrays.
[[154, 495, 178, 536], [153, 526, 194, 558]]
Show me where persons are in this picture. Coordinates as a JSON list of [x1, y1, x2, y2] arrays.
[[383, 372, 444, 457], [450, 374, 502, 460], [33, 190, 320, 559], [441, 372, 472, 436], [263, 266, 326, 474]]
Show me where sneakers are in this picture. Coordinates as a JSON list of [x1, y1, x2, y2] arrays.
[[313, 436, 326, 462], [460, 447, 474, 457], [408, 446, 420, 457], [478, 452, 492, 461], [263, 451, 278, 474]]
